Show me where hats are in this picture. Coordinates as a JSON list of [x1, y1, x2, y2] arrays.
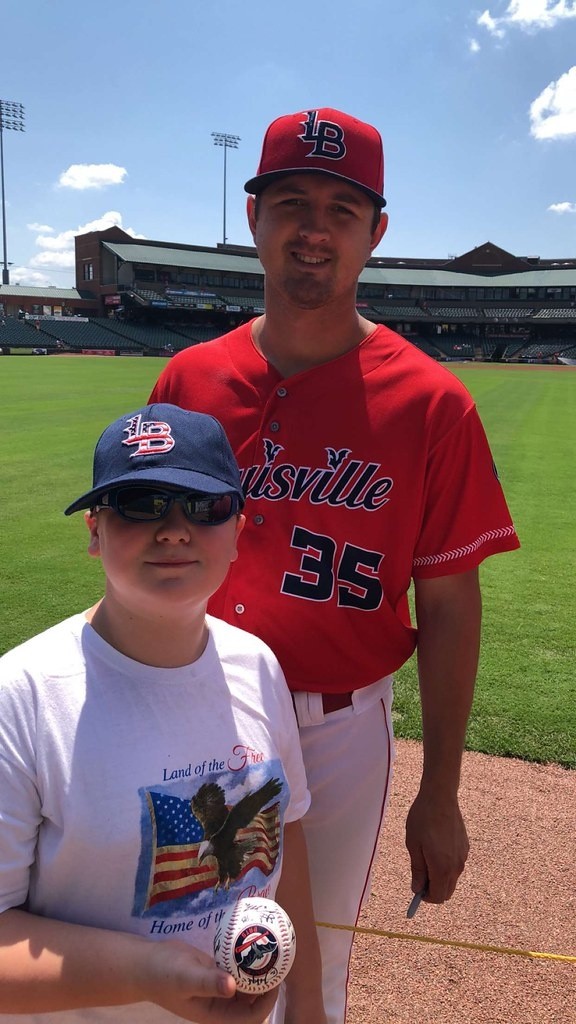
[[64, 404, 243, 517], [243, 107, 388, 207]]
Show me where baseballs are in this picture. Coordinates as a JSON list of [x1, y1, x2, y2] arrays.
[[208, 892, 299, 997]]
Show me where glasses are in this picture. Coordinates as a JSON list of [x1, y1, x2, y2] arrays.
[[91, 485, 241, 526]]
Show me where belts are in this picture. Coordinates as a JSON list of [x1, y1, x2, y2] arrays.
[[290, 688, 353, 722]]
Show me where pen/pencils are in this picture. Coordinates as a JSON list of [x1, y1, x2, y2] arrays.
[[407, 879, 429, 919]]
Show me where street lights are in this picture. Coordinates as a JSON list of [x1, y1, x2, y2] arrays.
[[210, 131, 242, 244], [0, 98, 27, 285]]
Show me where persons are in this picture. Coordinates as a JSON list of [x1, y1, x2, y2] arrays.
[[150, 107, 519, 1022], [0, 400, 326, 1024]]
